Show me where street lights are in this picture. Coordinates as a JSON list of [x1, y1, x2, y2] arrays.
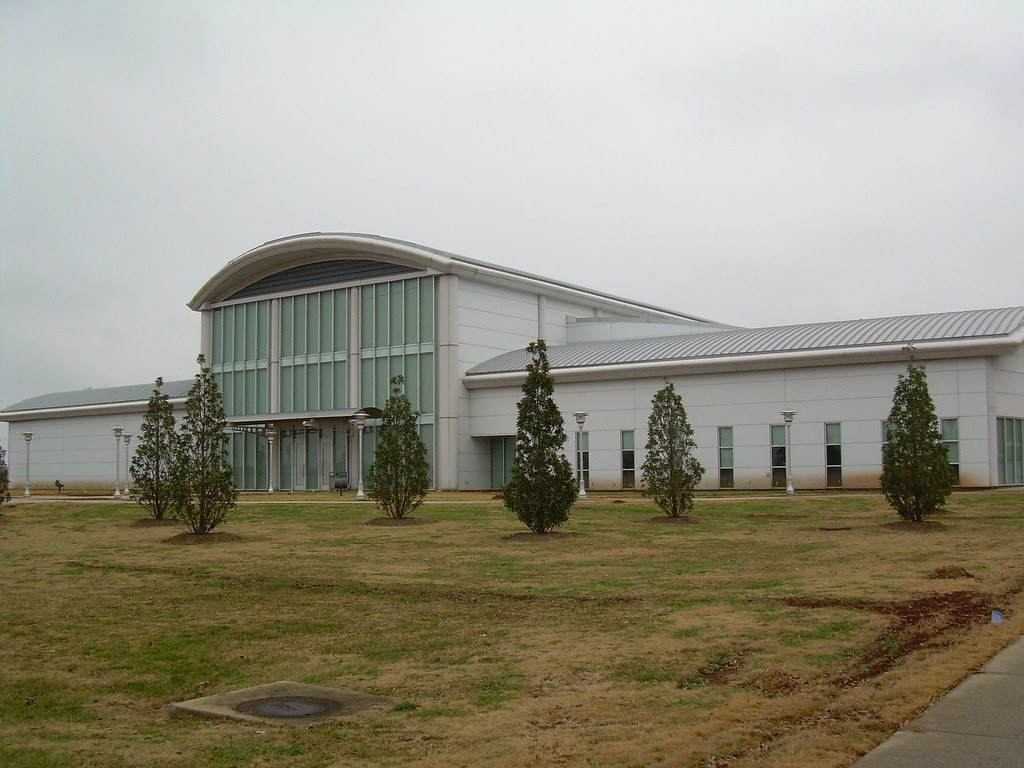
[[570, 410, 590, 496], [352, 410, 371, 497], [20, 430, 36, 497], [780, 408, 799, 494], [264, 426, 278, 494], [111, 423, 125, 498], [122, 433, 133, 495]]
